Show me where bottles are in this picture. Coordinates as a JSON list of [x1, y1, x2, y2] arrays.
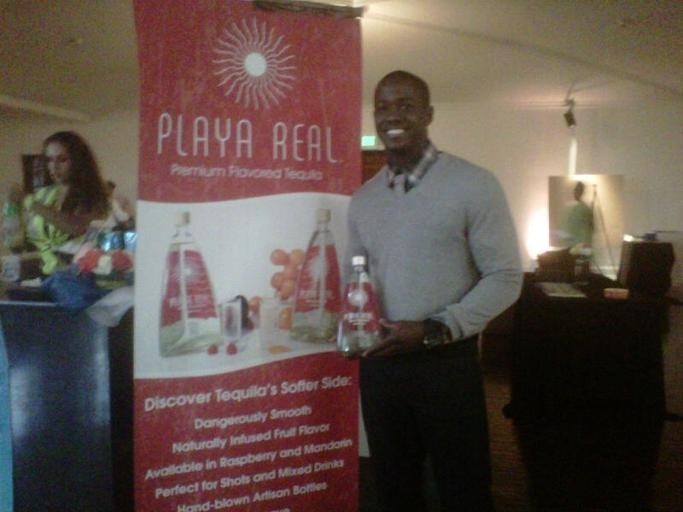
[[288, 206, 343, 345], [154, 213, 225, 359], [3, 189, 19, 240], [334, 253, 383, 359]]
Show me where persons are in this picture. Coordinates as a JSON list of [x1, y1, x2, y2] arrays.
[[88, 178, 130, 231], [8, 128, 110, 282], [559, 179, 593, 250], [334, 71, 522, 510]]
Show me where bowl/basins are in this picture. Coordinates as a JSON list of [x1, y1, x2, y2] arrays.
[[602, 287, 630, 301]]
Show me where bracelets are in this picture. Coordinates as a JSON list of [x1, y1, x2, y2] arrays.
[[420, 318, 457, 352]]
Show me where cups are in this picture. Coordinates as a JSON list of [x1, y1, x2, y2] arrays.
[[257, 296, 281, 349], [215, 299, 243, 349]]
